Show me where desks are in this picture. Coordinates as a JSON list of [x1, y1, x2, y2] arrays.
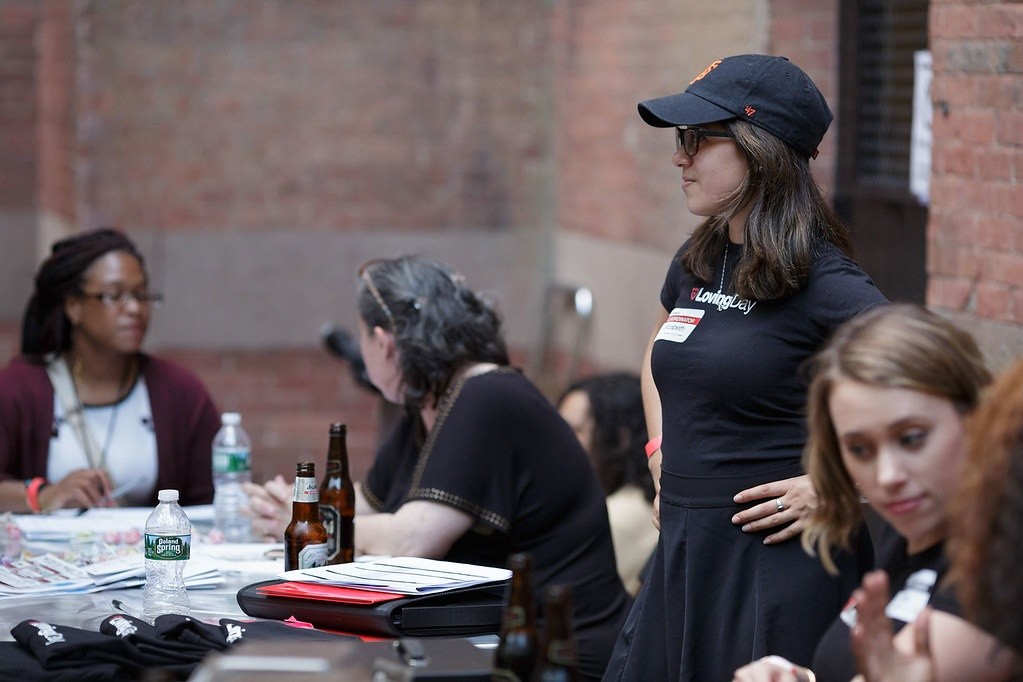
[[0, 501, 497, 682]]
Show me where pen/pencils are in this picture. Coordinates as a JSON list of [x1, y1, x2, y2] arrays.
[[77, 479, 143, 517]]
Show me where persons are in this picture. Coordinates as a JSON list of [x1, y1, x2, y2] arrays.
[[0, 229, 220, 515], [604, 53, 895, 681], [237, 258, 632, 682], [556, 372, 660, 597], [732, 304, 1023, 682], [851, 364, 1023, 682]]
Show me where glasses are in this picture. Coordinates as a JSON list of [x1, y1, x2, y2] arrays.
[[76, 289, 163, 310], [675, 127, 726, 156], [360, 254, 430, 342]]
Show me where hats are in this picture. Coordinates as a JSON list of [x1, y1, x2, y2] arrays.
[[635, 53, 835, 161]]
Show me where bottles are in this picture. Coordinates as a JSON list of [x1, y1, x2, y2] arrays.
[[491, 552, 584, 682], [142, 489, 192, 626], [284, 462, 329, 572], [209, 411, 253, 543], [319, 422, 356, 566]]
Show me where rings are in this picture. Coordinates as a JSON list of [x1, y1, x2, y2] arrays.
[[777, 498, 783, 511]]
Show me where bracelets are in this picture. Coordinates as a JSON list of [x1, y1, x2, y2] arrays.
[[25, 477, 48, 512], [803, 668, 816, 682]]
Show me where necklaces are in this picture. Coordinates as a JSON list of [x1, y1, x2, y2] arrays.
[[718, 244, 736, 311], [644, 436, 662, 456], [71, 362, 130, 496]]
